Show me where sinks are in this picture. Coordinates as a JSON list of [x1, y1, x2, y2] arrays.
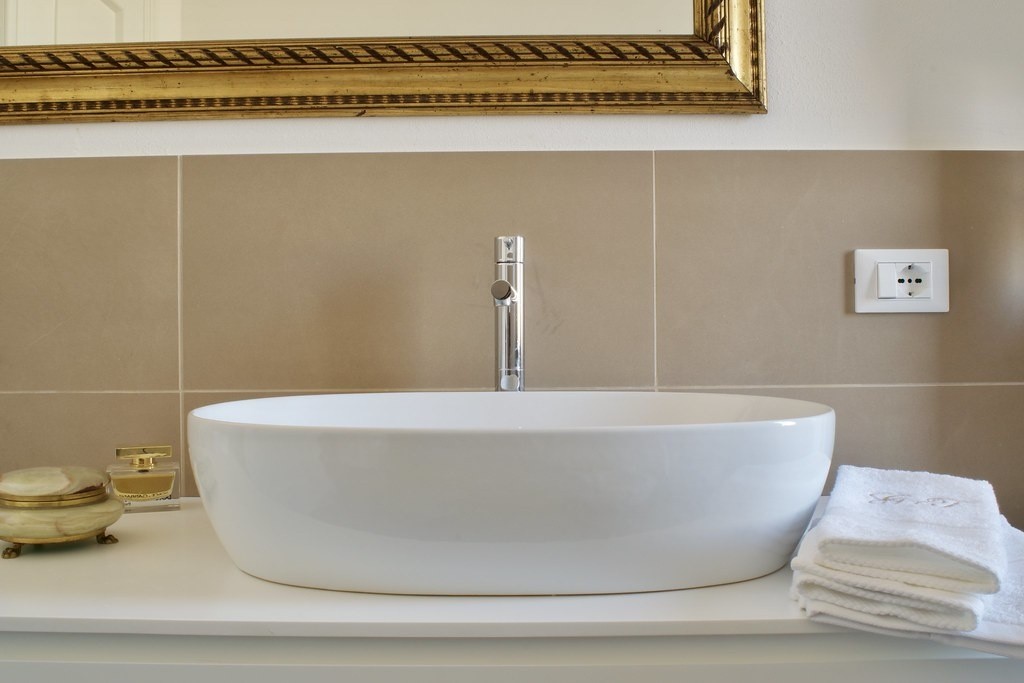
[[185, 387, 839, 600]]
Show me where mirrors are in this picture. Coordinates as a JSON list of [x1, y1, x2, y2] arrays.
[[0, 0, 769, 125]]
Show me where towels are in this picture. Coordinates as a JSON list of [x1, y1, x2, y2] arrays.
[[787, 459, 1023, 662]]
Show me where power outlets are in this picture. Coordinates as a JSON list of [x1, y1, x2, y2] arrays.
[[854, 249, 949, 313]]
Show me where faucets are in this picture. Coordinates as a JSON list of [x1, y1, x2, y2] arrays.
[[488, 232, 528, 394]]
